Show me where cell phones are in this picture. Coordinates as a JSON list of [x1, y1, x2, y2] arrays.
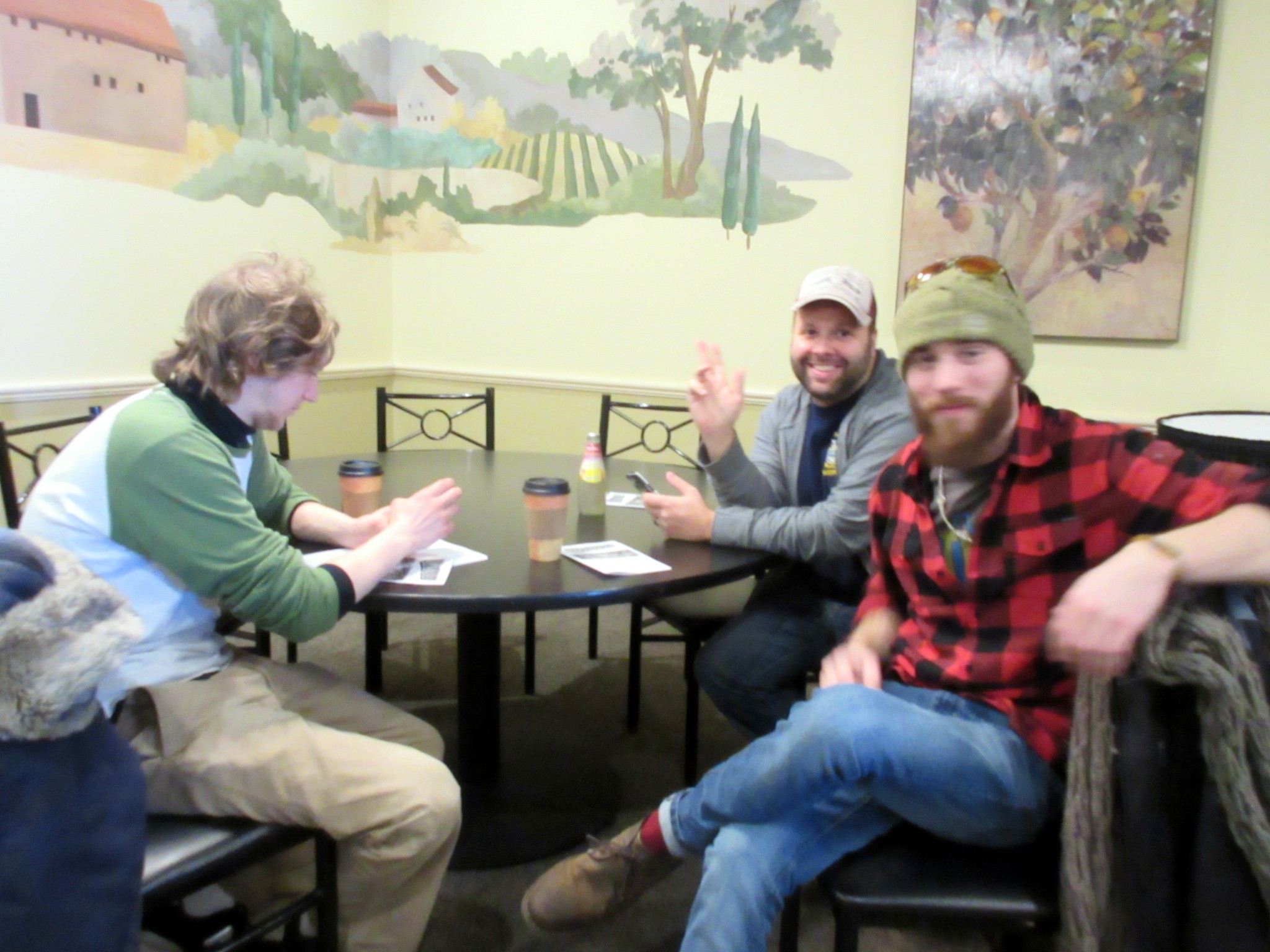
[[627, 471, 659, 494]]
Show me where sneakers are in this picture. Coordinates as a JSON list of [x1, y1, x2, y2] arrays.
[[520, 817, 679, 935]]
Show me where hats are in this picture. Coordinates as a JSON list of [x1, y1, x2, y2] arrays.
[[894, 259, 1033, 384], [793, 267, 876, 326]]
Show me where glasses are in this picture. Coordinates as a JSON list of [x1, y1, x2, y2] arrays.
[[903, 255, 1017, 295]]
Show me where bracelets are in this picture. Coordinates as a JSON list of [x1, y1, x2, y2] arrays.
[[1129, 531, 1187, 584]]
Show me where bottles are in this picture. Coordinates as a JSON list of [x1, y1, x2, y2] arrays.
[[578, 433, 605, 516]]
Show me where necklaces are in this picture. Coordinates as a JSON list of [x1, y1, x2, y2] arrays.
[[934, 465, 981, 544]]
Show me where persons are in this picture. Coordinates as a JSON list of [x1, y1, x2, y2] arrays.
[[519, 235, 1270, 952], [641, 268, 919, 739], [14, 254, 464, 952]]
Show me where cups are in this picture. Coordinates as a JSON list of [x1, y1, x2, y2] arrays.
[[338, 460, 385, 518], [523, 477, 571, 561]]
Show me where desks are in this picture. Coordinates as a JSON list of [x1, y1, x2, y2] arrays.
[[280, 449, 773, 821], [1157, 411, 1270, 468]]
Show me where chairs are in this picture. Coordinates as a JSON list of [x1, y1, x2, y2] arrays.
[[588, 395, 710, 659], [0, 407, 338, 952], [832, 681, 1206, 952], [365, 387, 536, 696], [628, 574, 757, 790]]
